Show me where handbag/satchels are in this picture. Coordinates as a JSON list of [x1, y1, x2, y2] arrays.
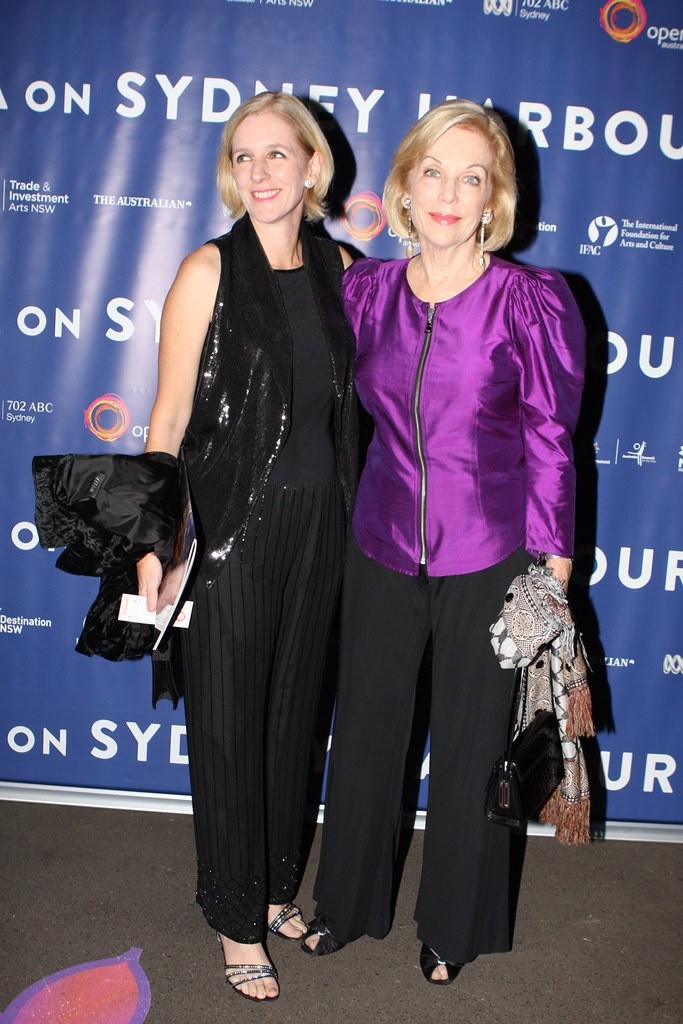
[[484, 710, 565, 831], [150, 629, 183, 710]]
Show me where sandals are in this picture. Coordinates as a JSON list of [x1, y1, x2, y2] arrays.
[[299, 916, 346, 958], [216, 932, 281, 1002], [420, 942, 465, 985], [267, 902, 308, 940]]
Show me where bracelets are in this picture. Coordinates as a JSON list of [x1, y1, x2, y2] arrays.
[[540, 553, 572, 563]]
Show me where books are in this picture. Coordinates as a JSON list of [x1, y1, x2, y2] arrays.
[[150, 451, 200, 653]]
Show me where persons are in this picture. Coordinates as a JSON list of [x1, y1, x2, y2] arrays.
[[136, 91, 372, 1003], [300, 99, 586, 985]]
